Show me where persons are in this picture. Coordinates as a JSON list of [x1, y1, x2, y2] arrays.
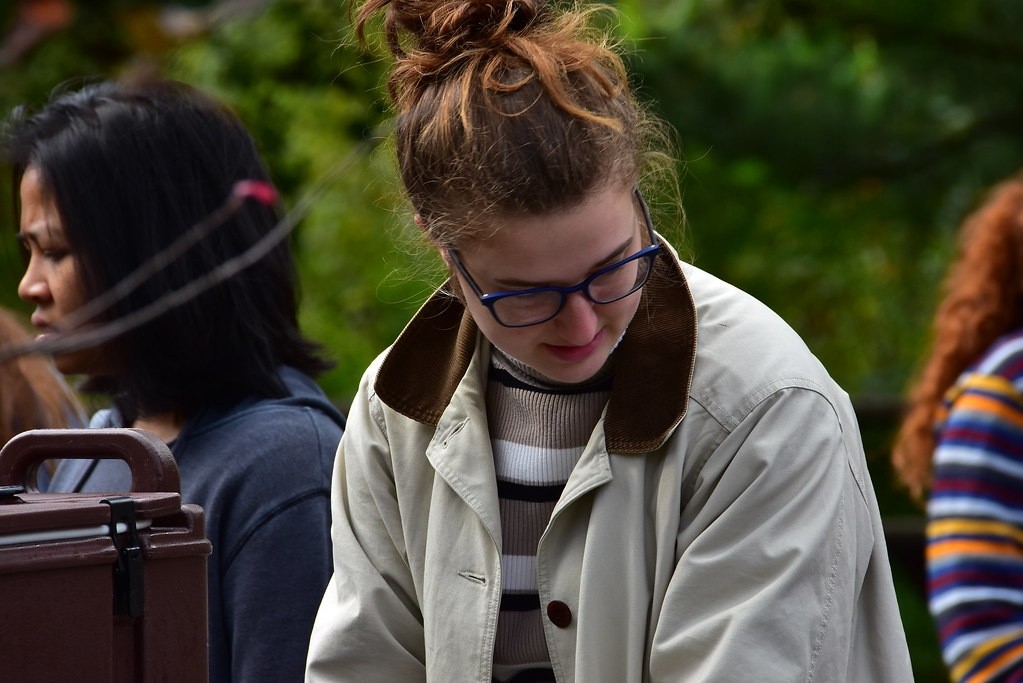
[[303, 0, 916, 683], [0, 78, 350, 683], [882, 179, 1023, 683]]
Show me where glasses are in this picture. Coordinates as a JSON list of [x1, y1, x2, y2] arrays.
[[447, 187, 660, 328]]
